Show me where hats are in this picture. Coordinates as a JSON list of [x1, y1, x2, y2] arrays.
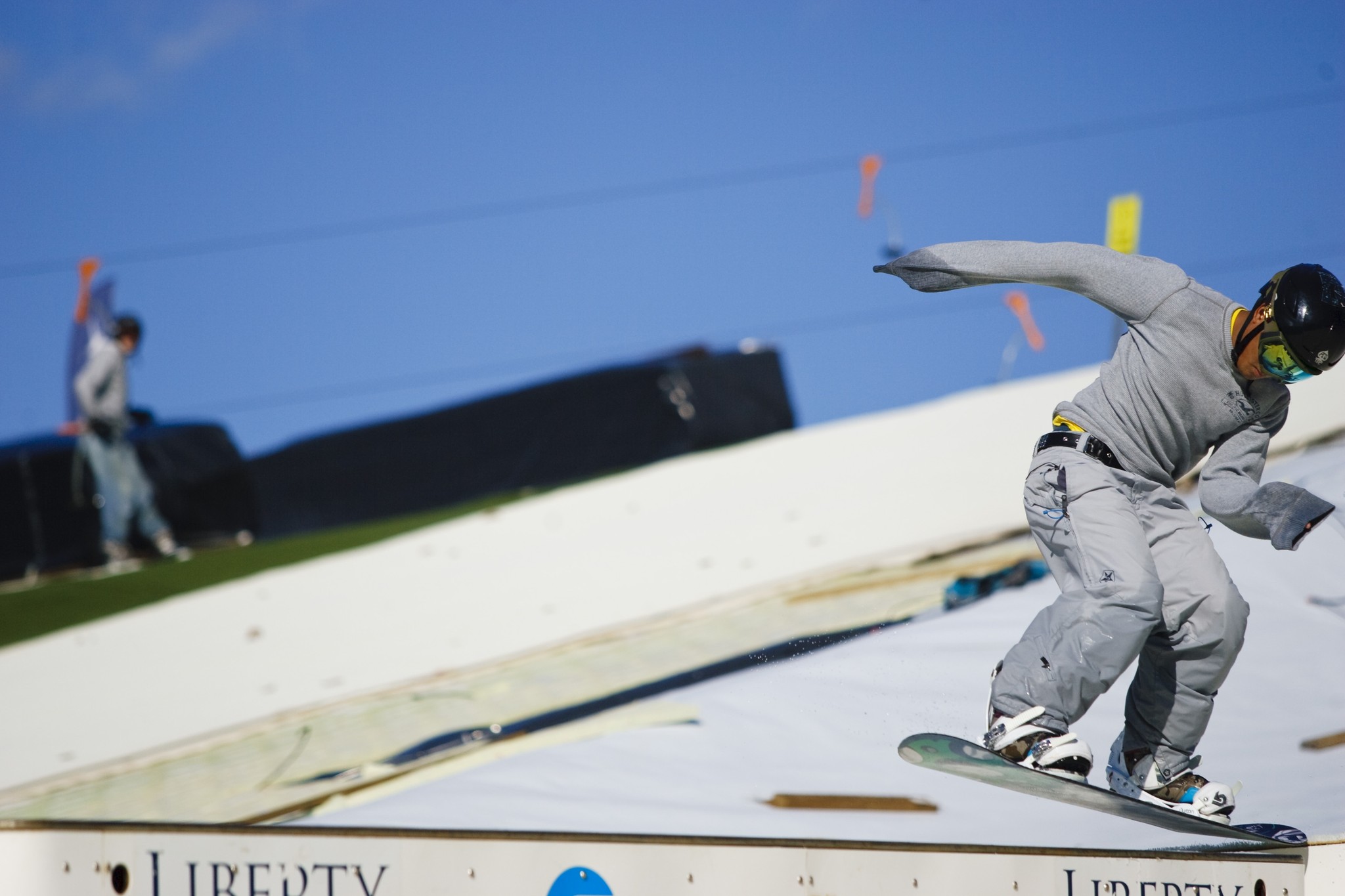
[[103, 310, 143, 343]]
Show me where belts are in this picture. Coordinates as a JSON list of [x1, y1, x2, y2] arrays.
[[1036, 434, 1117, 469]]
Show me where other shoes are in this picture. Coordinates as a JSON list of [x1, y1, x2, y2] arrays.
[[991, 706, 1063, 762], [1109, 749, 1213, 806], [100, 542, 146, 575], [147, 539, 195, 567]]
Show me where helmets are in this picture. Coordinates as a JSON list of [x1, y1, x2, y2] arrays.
[[1256, 262, 1345, 384]]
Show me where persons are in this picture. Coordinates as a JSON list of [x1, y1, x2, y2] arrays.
[[873, 240, 1345, 815], [75, 314, 177, 562]]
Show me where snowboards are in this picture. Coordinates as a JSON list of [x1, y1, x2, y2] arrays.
[[897, 730, 1311, 853]]
